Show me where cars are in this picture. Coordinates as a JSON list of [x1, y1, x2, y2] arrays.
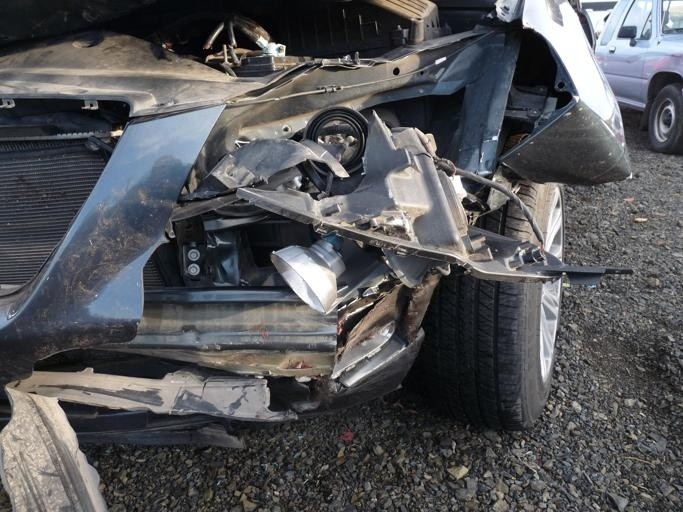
[[0, 0, 631, 510], [595, 1, 682, 156]]
